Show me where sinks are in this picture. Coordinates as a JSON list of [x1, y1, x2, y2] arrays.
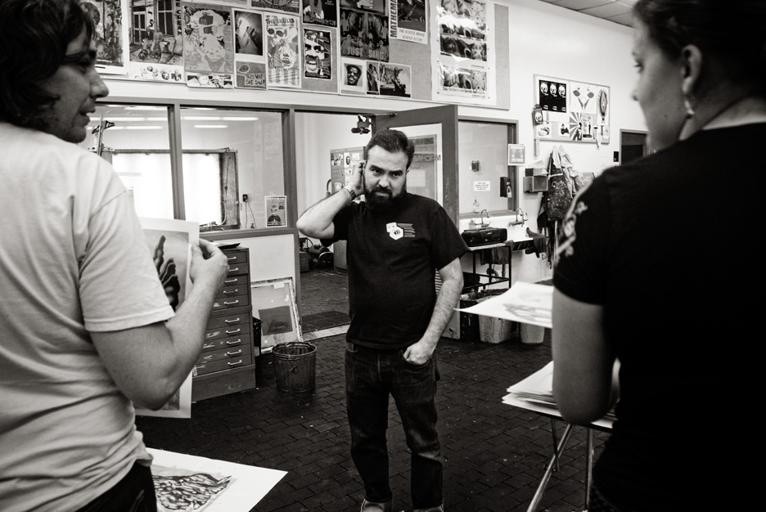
[[461, 228, 508, 247]]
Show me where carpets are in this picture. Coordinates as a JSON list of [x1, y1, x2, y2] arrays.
[[300, 309, 350, 334], [302, 310, 352, 333]]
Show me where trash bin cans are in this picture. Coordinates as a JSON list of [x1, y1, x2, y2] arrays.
[[299, 251, 313, 273]]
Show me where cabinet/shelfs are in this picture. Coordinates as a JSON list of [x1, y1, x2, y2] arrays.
[[192, 245, 257, 406]]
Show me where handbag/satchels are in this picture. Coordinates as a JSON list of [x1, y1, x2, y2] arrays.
[[537, 168, 595, 227]]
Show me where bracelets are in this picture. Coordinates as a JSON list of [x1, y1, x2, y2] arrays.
[[343, 186, 357, 201]]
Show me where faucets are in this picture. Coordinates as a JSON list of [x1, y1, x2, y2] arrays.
[[480, 207, 490, 228]]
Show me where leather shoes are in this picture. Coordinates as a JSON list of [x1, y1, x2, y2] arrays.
[[414, 504, 444, 512], [361, 499, 392, 512]]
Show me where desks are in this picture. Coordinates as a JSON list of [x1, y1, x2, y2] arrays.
[[501, 361, 615, 512], [464, 243, 512, 297]]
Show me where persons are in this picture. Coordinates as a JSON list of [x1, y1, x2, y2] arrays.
[[295, 128, 469, 511], [549, 1, 766, 511], [0, 1, 231, 511], [302, 1, 426, 99]]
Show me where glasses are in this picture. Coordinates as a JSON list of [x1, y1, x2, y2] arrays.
[[61, 50, 96, 72]]
